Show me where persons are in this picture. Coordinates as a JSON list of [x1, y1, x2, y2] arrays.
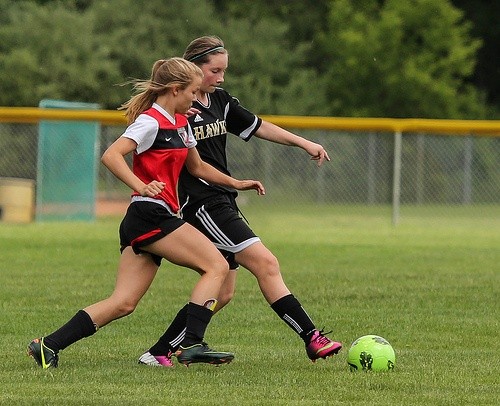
[[139, 35, 341, 369], [26, 56, 266, 370]]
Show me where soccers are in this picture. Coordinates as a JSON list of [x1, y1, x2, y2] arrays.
[[347, 334, 396, 374]]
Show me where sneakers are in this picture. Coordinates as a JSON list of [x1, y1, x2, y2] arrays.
[[305, 326, 342, 362], [27, 337, 59, 370], [138, 351, 181, 367], [177, 342, 234, 365]]
